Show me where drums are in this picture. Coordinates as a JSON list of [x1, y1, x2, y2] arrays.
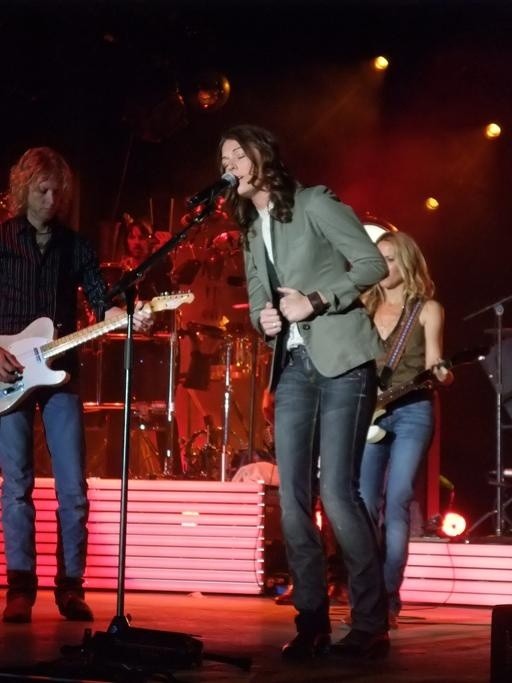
[[83, 399, 182, 478], [98, 261, 134, 302], [100, 332, 176, 404]]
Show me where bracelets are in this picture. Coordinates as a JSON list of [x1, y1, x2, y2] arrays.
[[307, 290, 328, 317]]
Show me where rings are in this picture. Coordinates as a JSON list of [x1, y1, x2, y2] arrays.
[[272, 322, 278, 328]]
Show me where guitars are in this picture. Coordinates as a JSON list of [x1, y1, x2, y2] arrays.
[[1, 289, 195, 414], [365, 339, 491, 443]]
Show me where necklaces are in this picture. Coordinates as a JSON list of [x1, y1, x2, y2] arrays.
[[376, 295, 397, 335], [36, 229, 52, 236]]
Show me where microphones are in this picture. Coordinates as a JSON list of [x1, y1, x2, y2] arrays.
[[186, 171, 239, 209]]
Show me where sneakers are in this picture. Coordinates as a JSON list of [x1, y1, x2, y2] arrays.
[[55, 592, 95, 621], [2, 590, 37, 622]]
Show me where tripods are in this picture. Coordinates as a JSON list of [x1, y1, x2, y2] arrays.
[[463, 295, 512, 538]]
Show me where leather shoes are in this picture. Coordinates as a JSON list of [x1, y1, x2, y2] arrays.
[[330, 625, 392, 661], [280, 628, 334, 661]]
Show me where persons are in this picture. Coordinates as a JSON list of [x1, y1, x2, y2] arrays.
[[213, 123, 394, 665], [267, 456, 347, 606], [339, 227, 455, 628], [0, 146, 157, 622], [91, 209, 203, 481]]
